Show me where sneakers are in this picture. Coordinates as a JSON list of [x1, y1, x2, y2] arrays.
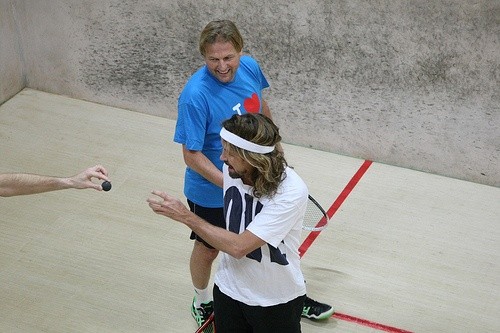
[[191, 296, 214, 333], [300, 296, 334, 320]]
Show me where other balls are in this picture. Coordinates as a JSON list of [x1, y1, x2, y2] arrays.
[[101, 181, 112, 191]]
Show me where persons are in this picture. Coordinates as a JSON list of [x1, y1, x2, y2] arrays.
[[1, 166, 111, 196], [174, 20, 333, 333], [146, 113, 308, 333]]
